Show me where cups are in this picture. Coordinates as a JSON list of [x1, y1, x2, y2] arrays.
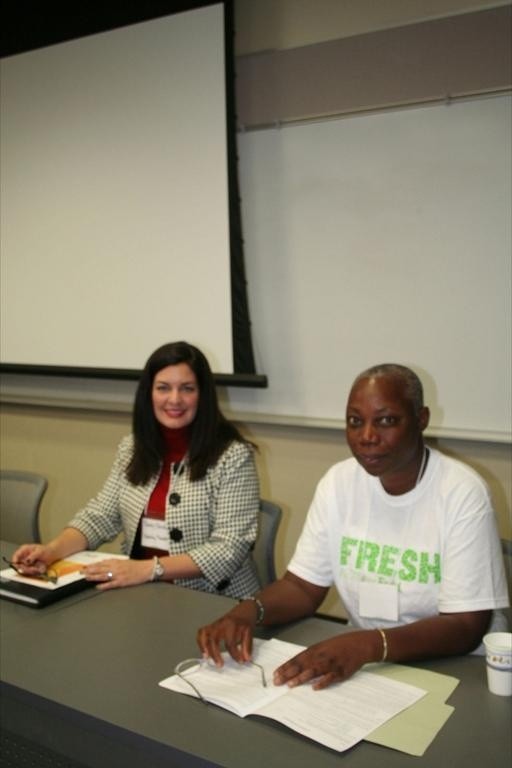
[[483, 632, 512, 697]]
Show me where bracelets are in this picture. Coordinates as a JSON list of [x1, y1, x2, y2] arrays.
[[238, 596, 265, 626], [374, 626, 388, 663]]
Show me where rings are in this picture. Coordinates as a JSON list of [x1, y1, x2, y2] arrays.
[[109, 571, 113, 580]]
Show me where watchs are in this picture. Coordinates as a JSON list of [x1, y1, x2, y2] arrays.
[[152, 554, 164, 583]]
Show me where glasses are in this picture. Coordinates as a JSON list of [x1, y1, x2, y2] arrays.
[[173, 655, 266, 702], [3, 555, 57, 583]]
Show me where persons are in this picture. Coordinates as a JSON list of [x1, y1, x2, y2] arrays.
[[195, 364, 511, 691], [12, 340, 262, 604]]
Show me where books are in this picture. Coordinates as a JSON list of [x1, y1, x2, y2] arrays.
[[1, 549, 133, 609]]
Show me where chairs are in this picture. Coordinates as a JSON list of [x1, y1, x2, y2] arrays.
[[0, 465, 282, 598]]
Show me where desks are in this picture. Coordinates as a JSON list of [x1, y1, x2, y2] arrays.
[[0, 539, 512, 768]]
